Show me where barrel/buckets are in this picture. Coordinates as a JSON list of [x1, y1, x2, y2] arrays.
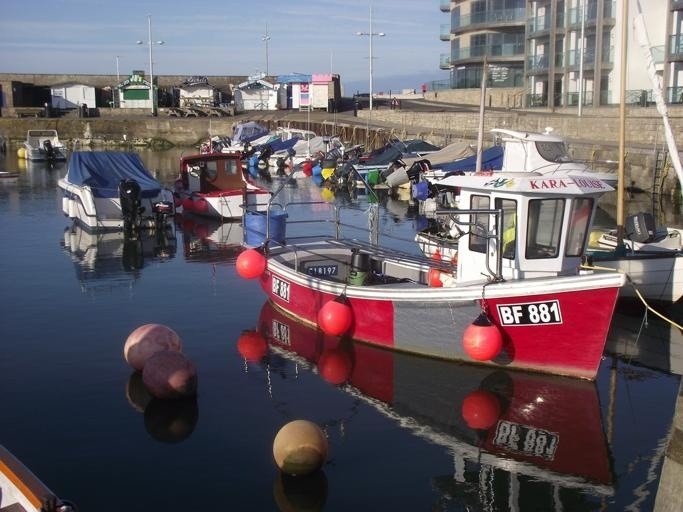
[[312, 177, 323, 185], [368, 189, 378, 204], [415, 216, 428, 233], [413, 181, 428, 200], [367, 172, 379, 186], [323, 188, 335, 203], [250, 156, 259, 167], [304, 161, 314, 176], [249, 167, 258, 177], [322, 168, 335, 180], [313, 165, 321, 175], [245, 208, 288, 249]]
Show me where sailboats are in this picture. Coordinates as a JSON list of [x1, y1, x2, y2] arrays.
[[412, 0, 683, 305]]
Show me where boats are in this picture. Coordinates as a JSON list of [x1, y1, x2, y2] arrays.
[[244, 297, 621, 511]]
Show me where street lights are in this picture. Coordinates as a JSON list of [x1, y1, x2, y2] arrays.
[[261, 23, 273, 82], [136, 11, 166, 117], [354, 5, 388, 111]]
